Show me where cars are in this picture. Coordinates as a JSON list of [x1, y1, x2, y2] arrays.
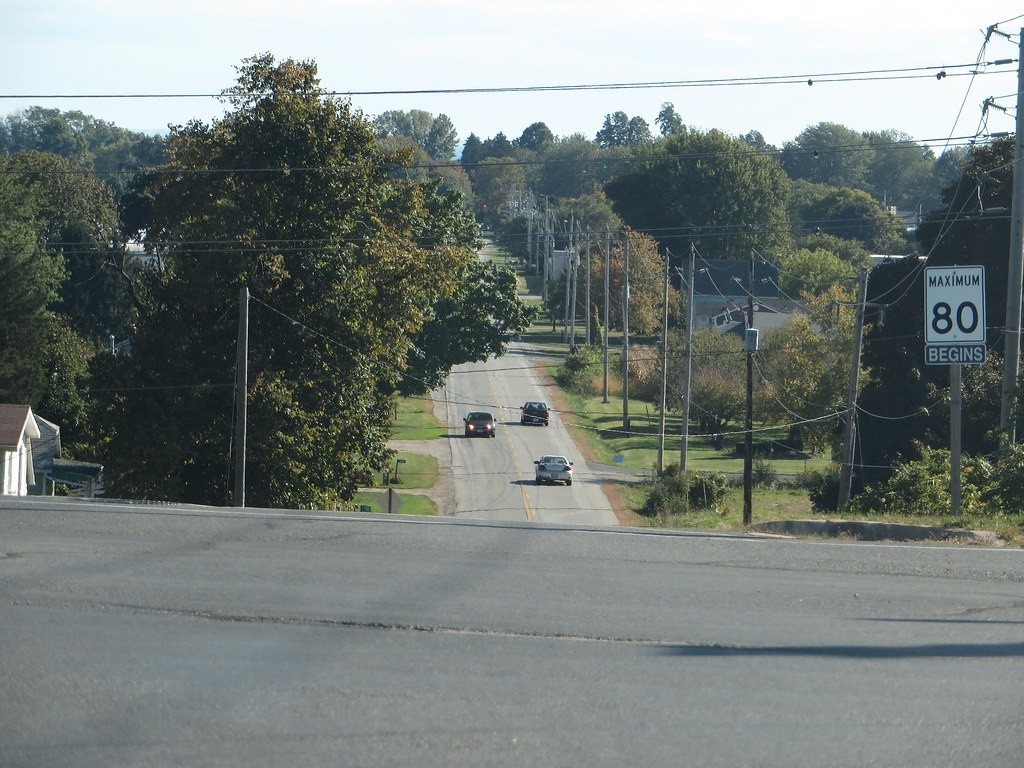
[[463, 412, 497, 438], [534, 455, 573, 486]]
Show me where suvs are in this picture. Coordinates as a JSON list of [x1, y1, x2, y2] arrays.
[[519, 402, 550, 426]]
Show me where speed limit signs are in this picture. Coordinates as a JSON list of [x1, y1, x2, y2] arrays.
[[926, 269, 984, 344]]
